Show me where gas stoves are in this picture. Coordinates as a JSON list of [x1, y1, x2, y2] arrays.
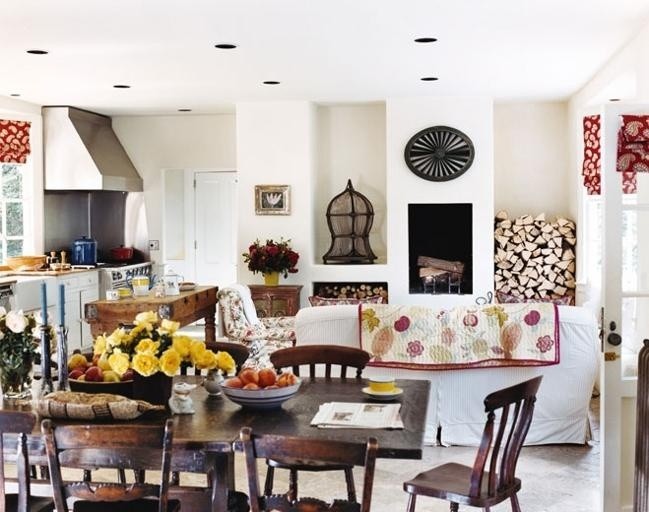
[[61, 257, 149, 282]]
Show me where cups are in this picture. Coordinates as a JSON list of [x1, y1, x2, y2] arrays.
[[118, 288, 130, 295], [369, 375, 396, 395], [105, 289, 119, 300], [132, 277, 150, 297]]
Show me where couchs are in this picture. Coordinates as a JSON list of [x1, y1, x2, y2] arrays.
[[219, 288, 601, 447]]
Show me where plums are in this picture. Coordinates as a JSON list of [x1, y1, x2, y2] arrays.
[[224, 367, 295, 390]]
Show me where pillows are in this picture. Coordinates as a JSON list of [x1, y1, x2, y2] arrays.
[[307, 295, 383, 305]]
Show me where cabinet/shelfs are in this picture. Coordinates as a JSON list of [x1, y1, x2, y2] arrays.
[[13, 271, 99, 371], [247, 285, 303, 317]]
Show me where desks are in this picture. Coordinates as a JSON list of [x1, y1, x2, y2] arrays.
[[84, 286, 220, 373], [2, 373, 433, 511]]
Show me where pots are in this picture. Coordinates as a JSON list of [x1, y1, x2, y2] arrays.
[[72, 235, 97, 264], [111, 245, 133, 261]]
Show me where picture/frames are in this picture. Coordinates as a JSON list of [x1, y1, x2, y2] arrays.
[[255, 185, 290, 216]]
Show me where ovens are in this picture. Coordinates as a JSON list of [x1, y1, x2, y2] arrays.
[[97, 280, 153, 299]]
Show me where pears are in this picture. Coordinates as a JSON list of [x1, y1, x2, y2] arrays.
[[77, 374, 85, 381], [97, 358, 110, 369], [103, 371, 119, 382], [68, 354, 87, 369]]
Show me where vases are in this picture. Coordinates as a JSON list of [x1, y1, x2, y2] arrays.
[[263, 272, 280, 286]]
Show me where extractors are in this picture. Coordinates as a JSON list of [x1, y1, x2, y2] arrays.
[[41, 105, 144, 193]]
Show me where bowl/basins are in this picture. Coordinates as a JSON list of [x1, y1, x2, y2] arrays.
[[216, 378, 303, 412], [50, 263, 71, 270], [5, 256, 47, 271]]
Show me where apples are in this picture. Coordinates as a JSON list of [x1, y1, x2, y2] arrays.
[[84, 367, 102, 382], [69, 370, 83, 378], [121, 371, 133, 381]]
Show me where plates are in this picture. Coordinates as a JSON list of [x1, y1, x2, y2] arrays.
[[178, 282, 198, 291], [361, 386, 406, 403]]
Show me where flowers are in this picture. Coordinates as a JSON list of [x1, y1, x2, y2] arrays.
[[241, 237, 298, 279]]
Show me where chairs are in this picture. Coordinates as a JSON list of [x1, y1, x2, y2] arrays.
[[2, 430, 55, 511], [177, 342, 251, 380], [237, 425, 380, 510], [37, 417, 176, 511], [402, 374, 542, 511], [262, 343, 373, 504]]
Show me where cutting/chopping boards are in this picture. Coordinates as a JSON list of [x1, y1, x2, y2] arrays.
[[5, 268, 85, 276]]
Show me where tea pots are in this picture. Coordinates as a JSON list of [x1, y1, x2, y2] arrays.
[[126, 273, 157, 292], [161, 269, 184, 296]]
